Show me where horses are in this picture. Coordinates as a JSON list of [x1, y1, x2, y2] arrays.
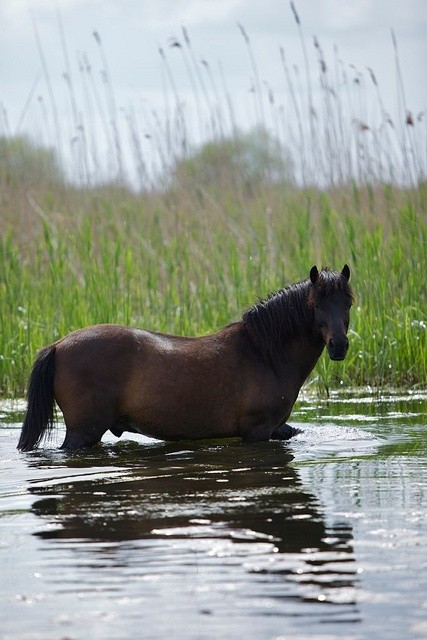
[[15, 264, 355, 453]]
